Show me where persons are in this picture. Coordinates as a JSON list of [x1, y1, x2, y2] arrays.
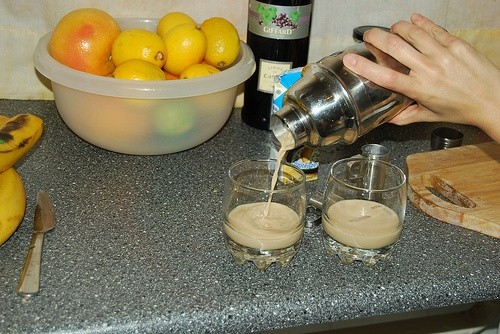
[[342, 13, 500, 145]]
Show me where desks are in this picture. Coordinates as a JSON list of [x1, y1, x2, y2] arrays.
[[0, 96, 500, 334]]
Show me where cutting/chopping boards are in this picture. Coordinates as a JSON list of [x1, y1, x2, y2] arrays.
[[405, 142, 500, 237]]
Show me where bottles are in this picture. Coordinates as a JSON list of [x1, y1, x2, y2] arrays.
[[241, 0, 313, 131], [269, 42, 414, 148]]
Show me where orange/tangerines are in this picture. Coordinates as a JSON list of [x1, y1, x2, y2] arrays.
[[49, 8, 122, 76]]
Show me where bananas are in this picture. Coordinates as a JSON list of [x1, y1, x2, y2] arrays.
[[0, 114, 44, 246]]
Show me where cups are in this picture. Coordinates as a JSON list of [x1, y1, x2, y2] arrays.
[[431, 128, 464, 151], [321, 157, 407, 267], [356, 144, 391, 200], [223, 159, 307, 270]]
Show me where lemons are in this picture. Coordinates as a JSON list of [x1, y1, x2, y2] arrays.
[[109, 12, 240, 137]]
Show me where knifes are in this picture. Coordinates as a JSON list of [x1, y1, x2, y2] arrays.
[[16, 190, 57, 296]]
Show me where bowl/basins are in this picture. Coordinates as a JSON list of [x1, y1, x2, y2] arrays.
[[33, 17, 256, 155]]
